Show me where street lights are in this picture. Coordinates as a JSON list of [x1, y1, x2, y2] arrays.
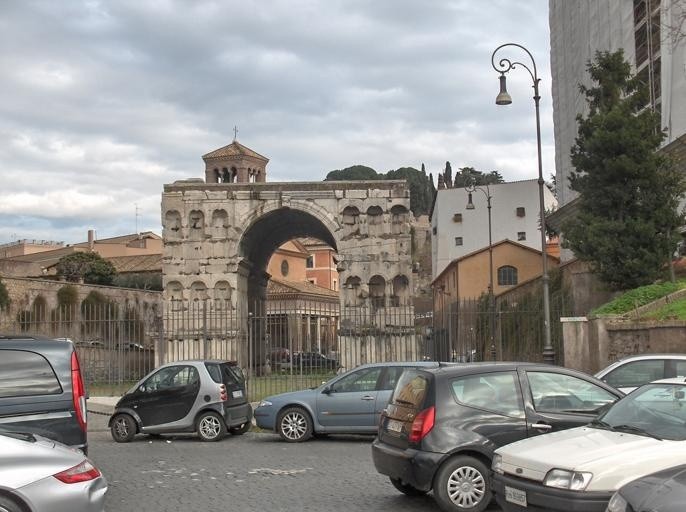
[[492, 43, 556, 365], [464, 175, 496, 361]]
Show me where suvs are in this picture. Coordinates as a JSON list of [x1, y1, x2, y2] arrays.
[[369, 361, 658, 512]]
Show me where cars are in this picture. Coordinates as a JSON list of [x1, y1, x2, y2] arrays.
[[254, 361, 458, 443], [0, 430, 108, 512], [268, 348, 335, 369], [486, 376, 685, 512], [531, 352, 686, 409], [108, 360, 252, 443], [605, 464, 685, 512]]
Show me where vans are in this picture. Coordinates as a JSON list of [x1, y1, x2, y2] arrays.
[[0, 334, 89, 457]]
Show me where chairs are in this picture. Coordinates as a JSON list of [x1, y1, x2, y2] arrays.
[[463, 382, 495, 408], [496, 387, 520, 413]]
